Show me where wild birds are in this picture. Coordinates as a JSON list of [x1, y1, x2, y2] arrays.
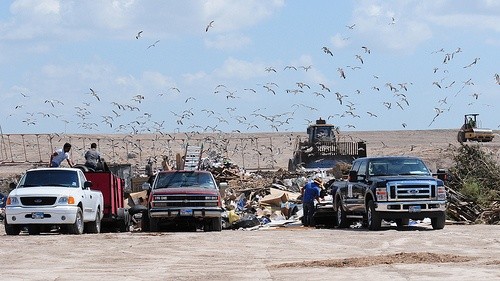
[[8, 16, 500, 164]]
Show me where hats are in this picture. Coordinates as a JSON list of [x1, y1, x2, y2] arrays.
[[314, 177, 323, 184]]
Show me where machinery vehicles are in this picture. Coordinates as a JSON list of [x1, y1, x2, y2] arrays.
[[288, 117, 355, 171], [457, 113, 495, 143]]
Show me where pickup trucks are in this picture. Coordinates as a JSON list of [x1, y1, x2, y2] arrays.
[[142, 170, 228, 231], [332, 156, 448, 232], [4, 168, 104, 235]]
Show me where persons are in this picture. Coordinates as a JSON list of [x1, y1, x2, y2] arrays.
[[301, 177, 326, 227], [468, 118, 474, 125], [84, 143, 100, 170], [50, 143, 74, 168], [61, 173, 76, 187]]
